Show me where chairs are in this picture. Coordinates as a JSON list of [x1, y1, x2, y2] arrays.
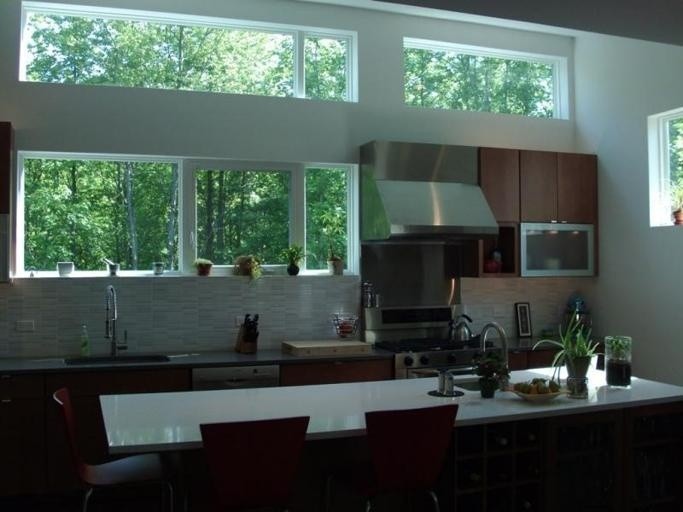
[[196, 414, 309, 512], [323, 402, 459, 511], [51, 386, 177, 512]]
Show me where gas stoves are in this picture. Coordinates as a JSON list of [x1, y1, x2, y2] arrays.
[[376, 336, 500, 370]]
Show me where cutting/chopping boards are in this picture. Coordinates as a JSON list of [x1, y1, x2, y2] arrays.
[[281, 339, 373, 358]]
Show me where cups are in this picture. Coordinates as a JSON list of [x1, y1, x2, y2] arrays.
[[57, 262, 73, 276], [107, 264, 119, 277]]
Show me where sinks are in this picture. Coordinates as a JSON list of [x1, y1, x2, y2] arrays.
[[453, 376, 505, 392], [63, 354, 172, 366]]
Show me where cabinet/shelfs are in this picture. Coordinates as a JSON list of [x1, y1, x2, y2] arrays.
[[0, 121, 15, 215], [517, 151, 599, 223], [441, 147, 521, 280], [0, 371, 47, 511], [277, 357, 393, 386], [508, 351, 553, 370]]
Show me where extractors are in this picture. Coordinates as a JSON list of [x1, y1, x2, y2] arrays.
[[359, 177, 498, 248]]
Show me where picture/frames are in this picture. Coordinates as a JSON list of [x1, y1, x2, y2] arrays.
[[514, 302, 532, 338]]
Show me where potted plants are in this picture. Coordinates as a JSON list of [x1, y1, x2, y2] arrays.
[[192, 257, 214, 275], [474, 348, 505, 390], [319, 210, 349, 274], [530, 311, 599, 402], [277, 243, 305, 275], [231, 253, 260, 286]]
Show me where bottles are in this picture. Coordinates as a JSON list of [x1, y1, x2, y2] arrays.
[[152, 262, 165, 276], [438, 370, 455, 395], [79, 324, 89, 358], [363, 281, 384, 307]]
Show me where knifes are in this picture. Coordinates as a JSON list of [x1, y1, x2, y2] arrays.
[[241, 313, 260, 343]]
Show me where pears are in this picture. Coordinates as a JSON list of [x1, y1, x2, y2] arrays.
[[513, 377, 560, 395]]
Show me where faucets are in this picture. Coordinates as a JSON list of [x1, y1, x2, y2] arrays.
[[103, 284, 129, 357], [480, 321, 511, 372]]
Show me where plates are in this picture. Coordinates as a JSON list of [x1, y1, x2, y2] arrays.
[[501, 385, 573, 405]]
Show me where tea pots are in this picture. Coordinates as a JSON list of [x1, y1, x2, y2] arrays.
[[448, 314, 474, 341]]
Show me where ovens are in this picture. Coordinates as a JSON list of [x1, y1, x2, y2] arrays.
[[394, 362, 490, 380]]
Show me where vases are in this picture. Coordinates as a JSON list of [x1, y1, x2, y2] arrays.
[[479, 385, 495, 399]]
